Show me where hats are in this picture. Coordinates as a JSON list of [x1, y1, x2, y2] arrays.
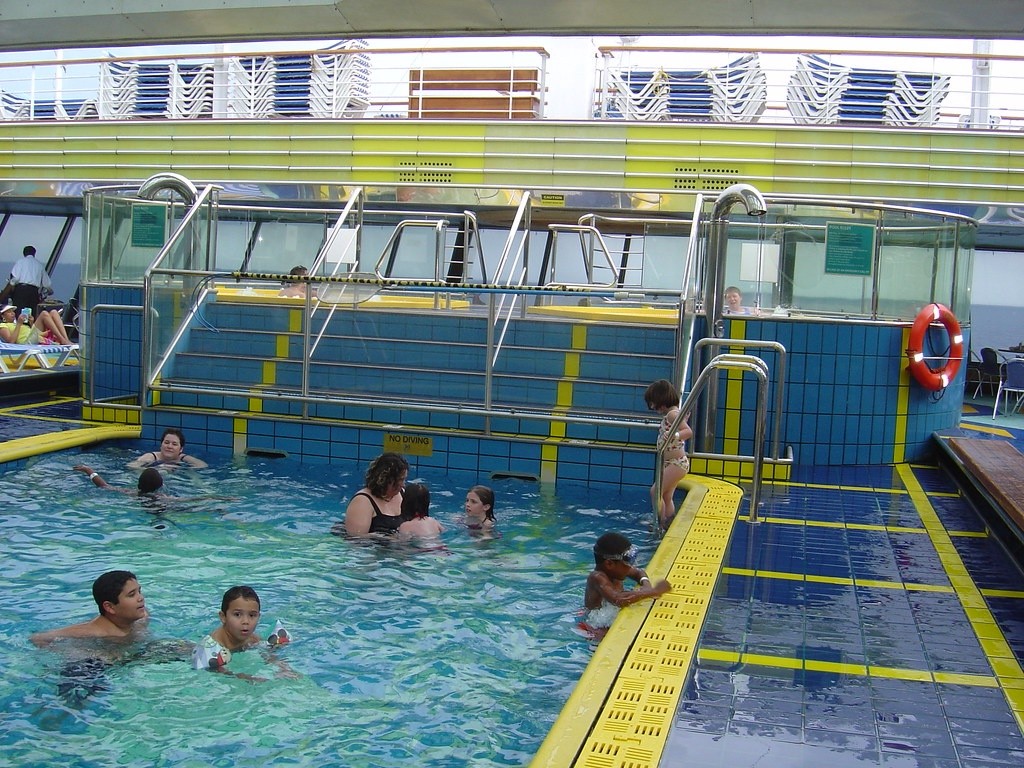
[[0, 305, 17, 313]]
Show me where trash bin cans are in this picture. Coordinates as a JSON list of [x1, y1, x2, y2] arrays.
[[37, 299, 65, 318]]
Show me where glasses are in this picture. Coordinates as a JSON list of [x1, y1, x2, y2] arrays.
[[620, 543, 640, 568]]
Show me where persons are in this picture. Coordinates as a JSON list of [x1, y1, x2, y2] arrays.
[[139, 427, 206, 466], [400, 483, 445, 536], [644, 379, 694, 523], [11, 246, 52, 327], [49, 569, 145, 637], [73, 464, 163, 493], [0, 304, 75, 345], [465, 485, 497, 525], [344, 452, 409, 534], [191, 586, 260, 668], [584, 531, 672, 608], [722, 286, 760, 316], [279, 264, 318, 297]]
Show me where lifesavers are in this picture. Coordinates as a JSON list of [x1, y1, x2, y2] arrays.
[[908, 304, 963, 390]]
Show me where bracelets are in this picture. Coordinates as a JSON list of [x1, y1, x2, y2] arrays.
[[90, 472, 99, 479], [640, 576, 649, 583]]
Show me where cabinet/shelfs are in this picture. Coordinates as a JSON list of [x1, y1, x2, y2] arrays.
[[408, 69, 550, 120]]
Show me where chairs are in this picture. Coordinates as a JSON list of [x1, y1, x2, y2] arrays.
[[972, 347, 1008, 398], [785, 52, 952, 127], [595, 53, 769, 123], [0, 38, 371, 122], [992, 358, 1024, 420], [962, 348, 984, 397], [0, 284, 80, 373]]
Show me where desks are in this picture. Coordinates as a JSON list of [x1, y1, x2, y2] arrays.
[[997, 348, 1024, 407]]
